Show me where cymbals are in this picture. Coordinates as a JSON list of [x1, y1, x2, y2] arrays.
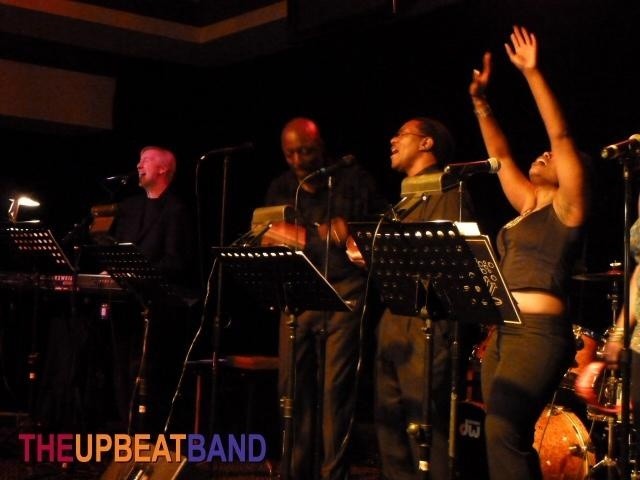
[[573, 268, 634, 280]]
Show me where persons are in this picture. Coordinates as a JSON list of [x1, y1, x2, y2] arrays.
[[467, 24, 597, 480], [32, 145, 195, 446], [344, 116, 479, 479], [258, 117, 395, 479], [600, 216, 640, 471]]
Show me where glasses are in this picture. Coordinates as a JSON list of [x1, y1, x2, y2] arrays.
[[396, 129, 425, 138]]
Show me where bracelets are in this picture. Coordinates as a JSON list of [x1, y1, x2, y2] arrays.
[[607, 326, 627, 343], [473, 103, 492, 118]]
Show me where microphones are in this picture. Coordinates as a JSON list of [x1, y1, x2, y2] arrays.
[[61, 212, 94, 244], [208, 141, 257, 156], [600, 133, 640, 160], [315, 154, 356, 176], [443, 158, 501, 179], [119, 170, 137, 185]]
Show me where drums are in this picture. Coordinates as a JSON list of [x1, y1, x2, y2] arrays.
[[459, 402, 596, 480], [468, 321, 607, 390]]
